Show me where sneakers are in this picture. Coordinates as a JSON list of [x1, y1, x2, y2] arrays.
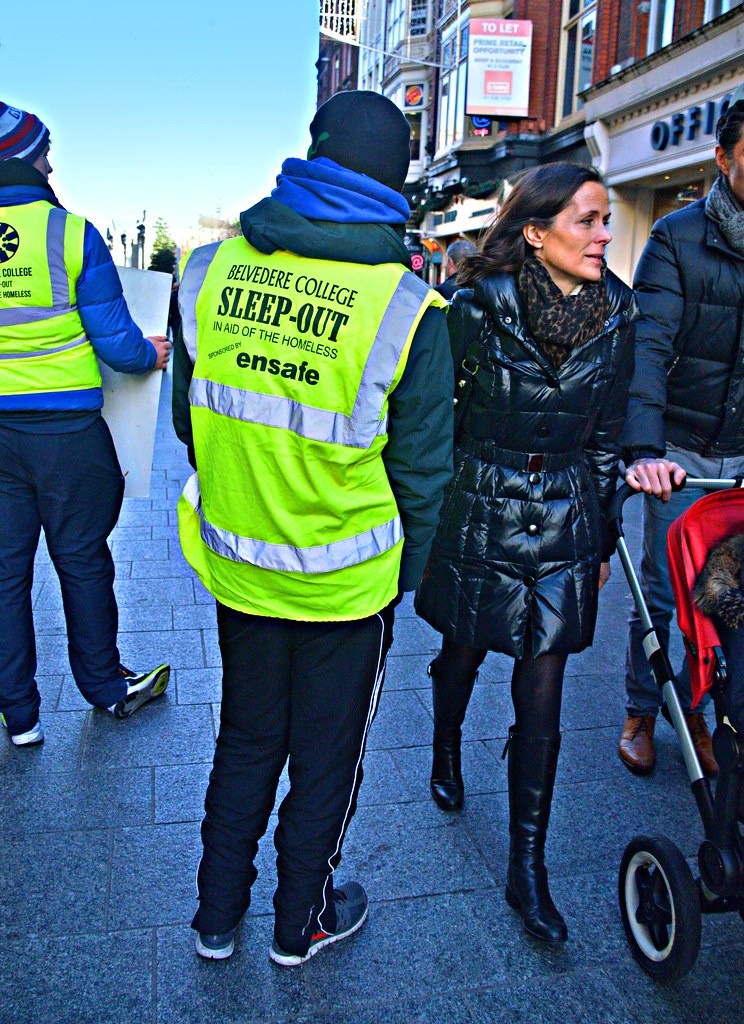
[[1, 713, 44, 747], [106, 662, 171, 720], [269, 881, 368, 967], [195, 857, 238, 960]]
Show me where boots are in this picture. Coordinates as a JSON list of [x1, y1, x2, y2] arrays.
[[426, 654, 480, 812], [501, 725, 569, 944]]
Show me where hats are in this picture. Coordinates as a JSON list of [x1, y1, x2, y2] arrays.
[[307, 90, 411, 193], [0, 102, 49, 166]]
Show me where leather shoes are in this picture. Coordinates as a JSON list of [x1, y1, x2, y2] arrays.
[[619, 713, 656, 775], [661, 701, 721, 777]]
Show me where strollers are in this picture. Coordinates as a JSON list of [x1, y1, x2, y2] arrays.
[[610, 472, 744, 987]]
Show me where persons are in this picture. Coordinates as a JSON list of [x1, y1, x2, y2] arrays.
[[412, 160, 635, 949], [614, 98, 744, 776], [163, 90, 456, 969], [0, 98, 171, 747]]
